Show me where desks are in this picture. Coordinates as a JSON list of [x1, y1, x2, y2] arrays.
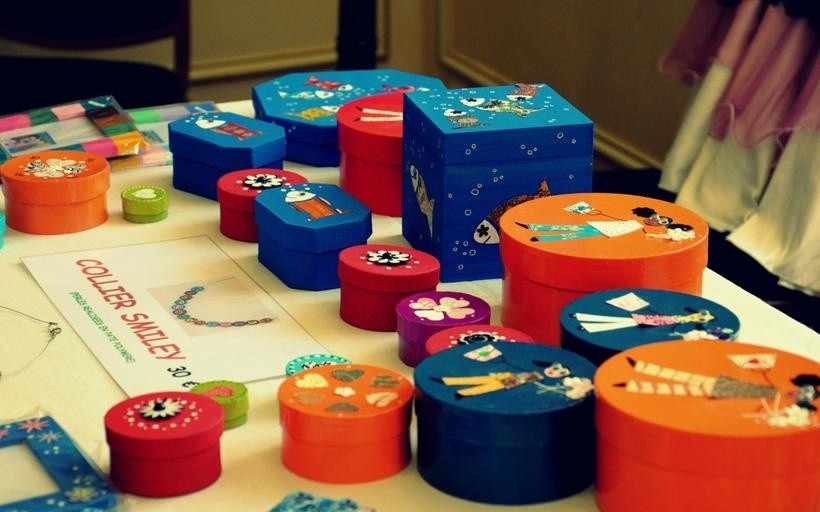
[[1, 92, 819, 512]]
